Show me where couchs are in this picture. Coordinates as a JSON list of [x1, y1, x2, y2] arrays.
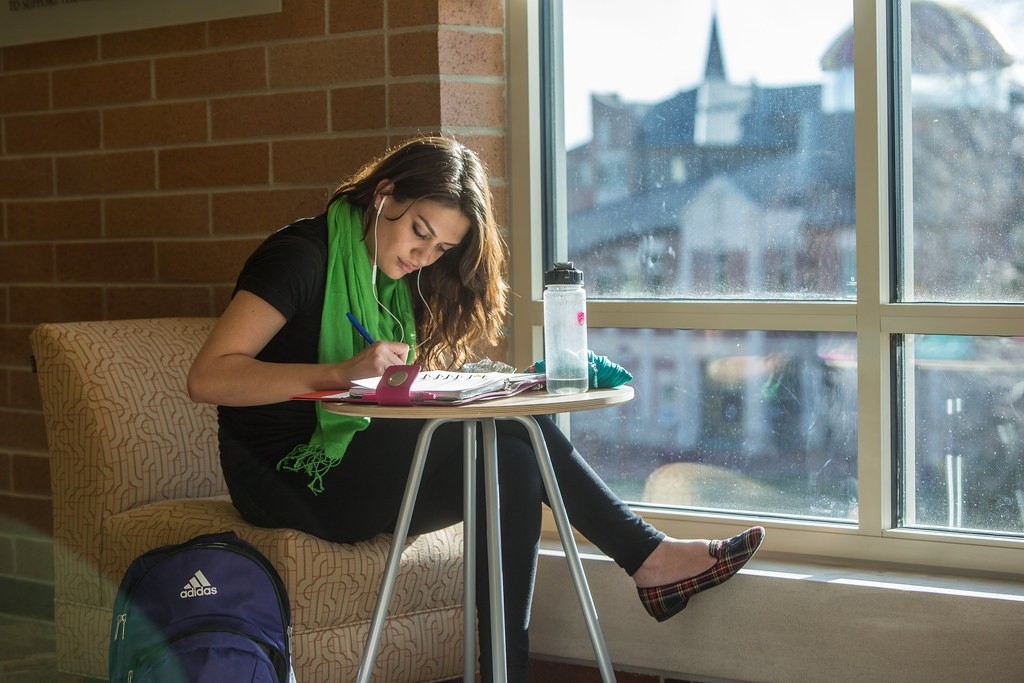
[[28, 316, 481, 683]]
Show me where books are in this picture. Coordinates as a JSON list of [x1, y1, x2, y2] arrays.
[[347, 362, 546, 405]]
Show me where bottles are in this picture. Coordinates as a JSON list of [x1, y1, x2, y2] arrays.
[[542, 260, 589, 396]]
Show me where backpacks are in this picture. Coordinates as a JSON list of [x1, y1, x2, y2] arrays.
[[108, 532, 297, 683]]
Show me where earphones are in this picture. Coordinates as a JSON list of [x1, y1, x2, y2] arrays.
[[378, 194, 388, 216]]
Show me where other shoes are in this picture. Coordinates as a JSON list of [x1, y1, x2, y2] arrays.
[[636, 526, 765, 623]]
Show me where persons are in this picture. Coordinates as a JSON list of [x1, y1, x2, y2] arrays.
[[186, 137, 766, 683]]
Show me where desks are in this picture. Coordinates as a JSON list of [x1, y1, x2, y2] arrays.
[[320, 385, 636, 683]]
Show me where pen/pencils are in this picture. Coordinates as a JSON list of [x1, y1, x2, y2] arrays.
[[345, 311, 374, 344]]
